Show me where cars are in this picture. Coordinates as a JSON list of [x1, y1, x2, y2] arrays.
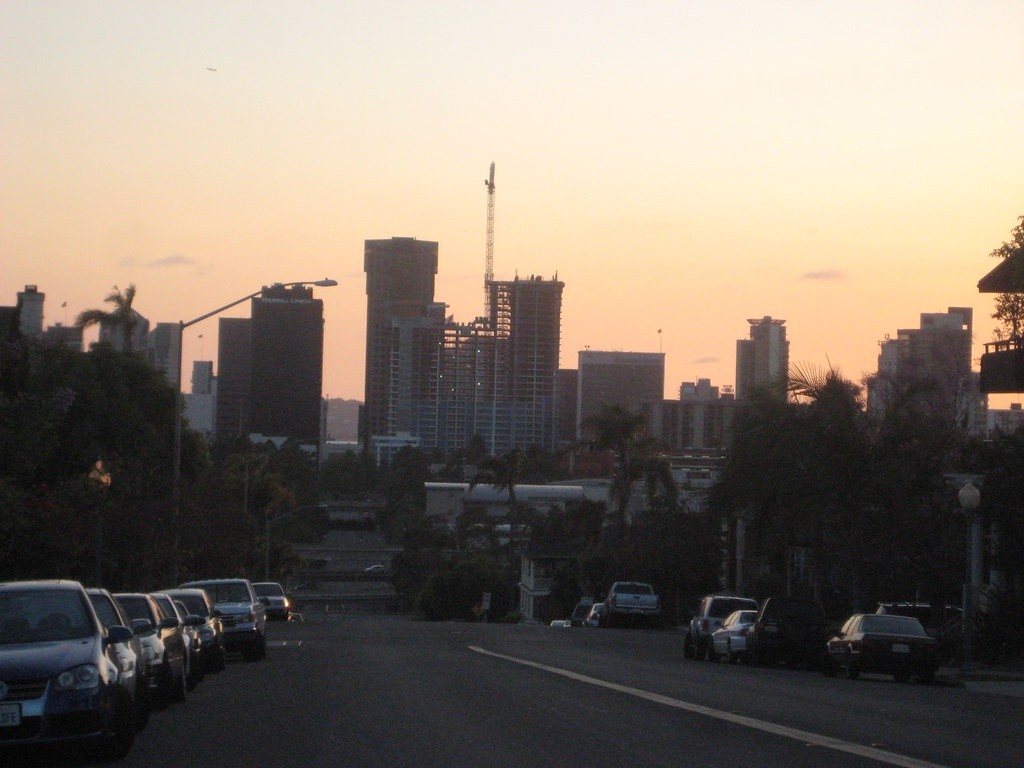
[[84, 585, 224, 733], [820, 612, 941, 681], [706, 610, 759, 664], [550, 599, 603, 629], [363, 564, 386, 574], [248, 583, 290, 622], [0, 579, 134, 768]]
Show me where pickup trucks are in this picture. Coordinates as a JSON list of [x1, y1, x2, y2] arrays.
[[600, 582, 660, 629]]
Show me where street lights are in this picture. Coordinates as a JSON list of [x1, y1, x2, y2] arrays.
[[169, 276, 339, 589], [241, 436, 337, 578], [265, 503, 328, 583]]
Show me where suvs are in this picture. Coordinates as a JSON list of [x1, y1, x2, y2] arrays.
[[745, 585, 853, 675], [683, 593, 760, 662], [178, 578, 267, 661]]
